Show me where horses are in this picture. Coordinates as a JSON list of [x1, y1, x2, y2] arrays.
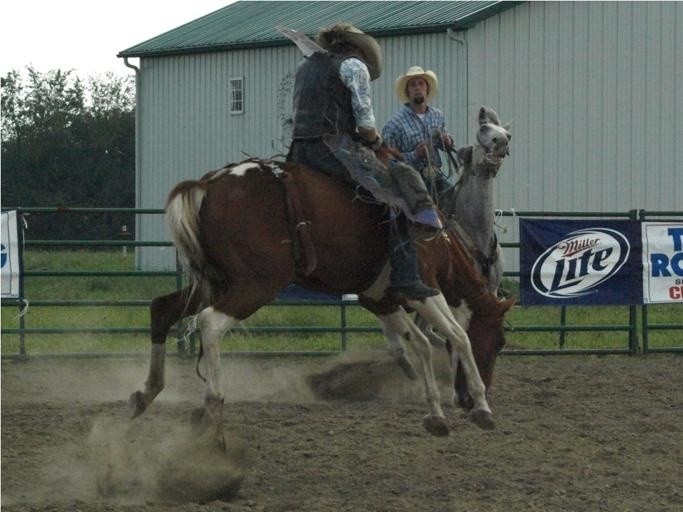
[[128, 158, 517, 455], [387, 105, 515, 382]]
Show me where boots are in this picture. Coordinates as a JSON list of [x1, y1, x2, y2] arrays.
[[387, 226, 438, 300]]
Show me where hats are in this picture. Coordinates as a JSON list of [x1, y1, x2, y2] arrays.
[[319, 23, 383, 82], [394, 66, 437, 102]]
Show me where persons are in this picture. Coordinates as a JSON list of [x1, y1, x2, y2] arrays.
[[286, 21, 443, 299], [380, 65, 454, 213]]
